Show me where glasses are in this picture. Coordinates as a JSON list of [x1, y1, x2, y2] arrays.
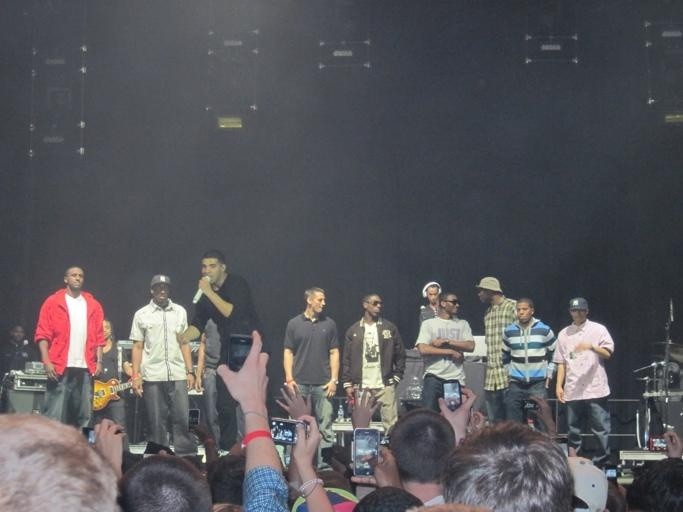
[[366, 300, 383, 306], [445, 299, 461, 305]]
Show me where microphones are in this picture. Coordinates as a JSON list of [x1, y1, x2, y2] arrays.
[[193, 274, 211, 305]]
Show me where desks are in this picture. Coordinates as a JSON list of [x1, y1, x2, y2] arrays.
[[331, 420, 385, 451], [618, 448, 668, 488]]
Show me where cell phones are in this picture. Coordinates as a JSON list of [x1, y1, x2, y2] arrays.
[[186, 409, 200, 434], [226, 334, 253, 374], [604, 465, 620, 489], [443, 379, 463, 412], [81, 426, 124, 445], [351, 428, 380, 478]]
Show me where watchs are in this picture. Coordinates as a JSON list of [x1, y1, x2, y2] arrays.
[[331, 378, 339, 386]]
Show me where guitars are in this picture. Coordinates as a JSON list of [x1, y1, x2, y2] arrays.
[[93, 379, 144, 410]]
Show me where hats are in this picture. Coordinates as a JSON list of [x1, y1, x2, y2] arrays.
[[475, 277, 504, 293], [422, 281, 442, 298], [566, 456, 609, 512], [150, 274, 171, 289], [568, 297, 589, 311]]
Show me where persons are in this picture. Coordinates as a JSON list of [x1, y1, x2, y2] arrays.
[[346, 385, 383, 433], [341, 293, 407, 431], [33, 266, 106, 435], [388, 410, 456, 507], [501, 297, 556, 433], [275, 379, 322, 504], [624, 430, 683, 511], [282, 286, 340, 430], [415, 292, 476, 412], [469, 409, 488, 442], [85, 417, 126, 481], [522, 395, 559, 442], [419, 281, 441, 325], [0, 324, 31, 371], [129, 274, 194, 455], [435, 382, 477, 444], [116, 454, 213, 512], [216, 328, 290, 511], [552, 298, 620, 481], [442, 422, 574, 512], [475, 277, 517, 427], [0, 412, 117, 512], [208, 455, 246, 504], [177, 250, 263, 458]]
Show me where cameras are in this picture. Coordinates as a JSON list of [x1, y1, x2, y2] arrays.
[[270, 418, 307, 446], [650, 435, 671, 454]]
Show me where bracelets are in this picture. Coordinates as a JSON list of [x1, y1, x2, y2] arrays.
[[444, 338, 450, 346], [287, 379, 293, 385], [240, 429, 272, 448], [185, 369, 194, 375], [296, 477, 325, 494]]
